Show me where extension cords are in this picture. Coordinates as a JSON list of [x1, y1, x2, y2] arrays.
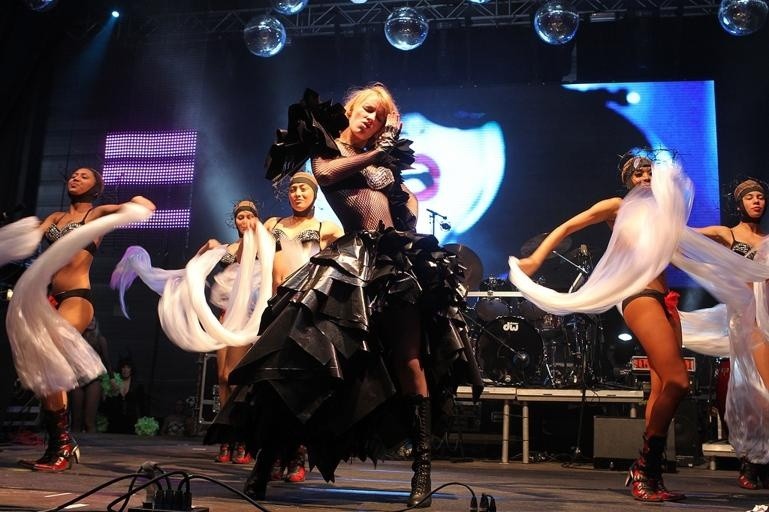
[[127, 505, 209, 512]]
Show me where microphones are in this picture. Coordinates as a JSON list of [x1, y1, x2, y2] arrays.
[[580, 244, 589, 267]]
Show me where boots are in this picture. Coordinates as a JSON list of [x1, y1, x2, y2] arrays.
[[245, 447, 270, 501], [216, 443, 250, 464], [271, 444, 305, 482], [408, 392, 434, 508], [19, 406, 80, 472], [737, 459, 760, 489], [625, 430, 688, 502]]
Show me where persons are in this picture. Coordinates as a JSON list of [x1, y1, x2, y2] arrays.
[[245, 81, 432, 507], [195, 201, 258, 464], [19, 168, 157, 473], [688, 179, 769, 489], [518, 157, 690, 501], [110, 357, 146, 433], [159, 397, 193, 440], [248, 172, 340, 482], [72, 315, 109, 431]]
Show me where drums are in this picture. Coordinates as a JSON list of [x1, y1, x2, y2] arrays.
[[475, 279, 513, 322], [518, 301, 561, 342], [477, 316, 544, 384]]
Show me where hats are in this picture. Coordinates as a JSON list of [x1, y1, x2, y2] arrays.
[[621, 156, 652, 185], [734, 179, 764, 203], [234, 201, 258, 217], [92, 169, 105, 195], [287, 172, 318, 192]]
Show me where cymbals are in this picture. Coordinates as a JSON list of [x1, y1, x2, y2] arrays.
[[440, 242, 483, 285], [518, 232, 572, 259]]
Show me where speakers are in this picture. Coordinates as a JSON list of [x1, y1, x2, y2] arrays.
[[593, 415, 677, 474]]
